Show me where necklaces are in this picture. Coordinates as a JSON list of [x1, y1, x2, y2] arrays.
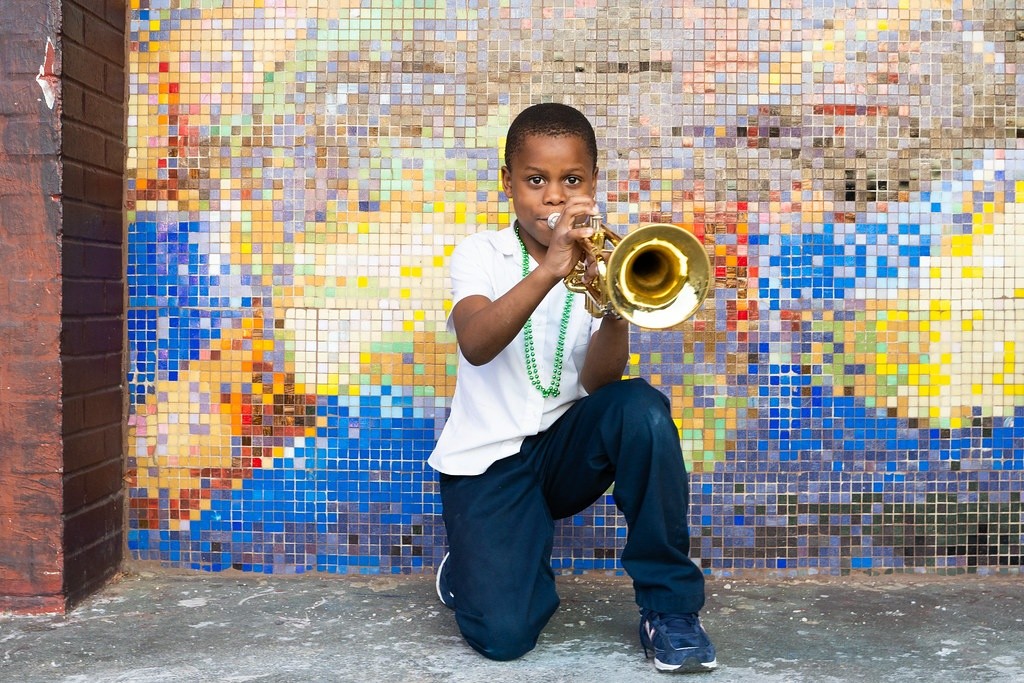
[[516, 226, 573, 397]]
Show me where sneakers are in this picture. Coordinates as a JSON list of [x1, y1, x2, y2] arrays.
[[436, 552, 455, 609], [638, 606, 717, 673]]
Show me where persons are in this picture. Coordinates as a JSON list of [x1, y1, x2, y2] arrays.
[[428, 103, 718, 672]]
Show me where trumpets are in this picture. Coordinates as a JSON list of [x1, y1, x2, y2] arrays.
[[547, 212, 712, 330]]
